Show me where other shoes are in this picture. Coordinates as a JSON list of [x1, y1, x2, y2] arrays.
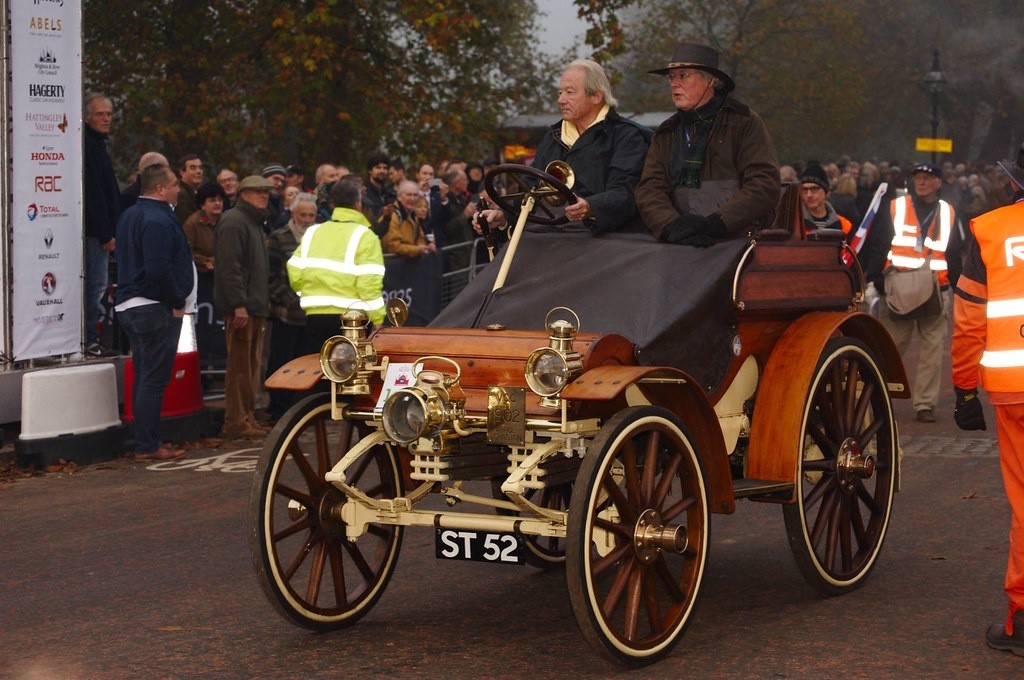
[[202, 379, 224, 393], [917, 409, 936, 422]]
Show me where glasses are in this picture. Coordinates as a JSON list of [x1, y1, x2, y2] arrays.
[[89, 111, 114, 119], [248, 189, 269, 195], [665, 72, 700, 83], [802, 186, 820, 194]]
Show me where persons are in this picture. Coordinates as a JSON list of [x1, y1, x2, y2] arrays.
[[865, 164, 963, 423], [83, 92, 121, 357], [122, 152, 506, 437], [802, 165, 869, 280], [780, 156, 919, 217], [115, 165, 185, 459], [634, 43, 781, 247], [472, 60, 654, 235], [952, 143, 1024, 658], [942, 159, 1024, 215]]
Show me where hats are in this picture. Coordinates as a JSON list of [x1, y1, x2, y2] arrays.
[[286, 165, 303, 175], [261, 163, 287, 177], [912, 163, 942, 178], [483, 159, 499, 166], [802, 164, 830, 193], [647, 43, 735, 93], [237, 175, 275, 195], [997, 143, 1024, 190]]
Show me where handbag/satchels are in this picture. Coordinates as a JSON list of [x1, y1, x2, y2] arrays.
[[884, 270, 943, 321]]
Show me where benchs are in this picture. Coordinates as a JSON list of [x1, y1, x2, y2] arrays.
[[753, 179, 846, 241]]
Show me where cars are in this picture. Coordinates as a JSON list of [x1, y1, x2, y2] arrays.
[[244, 160, 915, 671]]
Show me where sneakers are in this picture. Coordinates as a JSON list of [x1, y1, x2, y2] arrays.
[[987, 611, 1024, 657], [251, 421, 272, 433], [220, 425, 268, 439], [85, 340, 121, 359]]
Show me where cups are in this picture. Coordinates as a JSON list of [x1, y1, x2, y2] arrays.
[[425, 234, 435, 242]]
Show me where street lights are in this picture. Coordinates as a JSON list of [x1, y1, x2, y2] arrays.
[[919, 44, 950, 168]]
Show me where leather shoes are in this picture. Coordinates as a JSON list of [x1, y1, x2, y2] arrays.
[[135, 444, 186, 462]]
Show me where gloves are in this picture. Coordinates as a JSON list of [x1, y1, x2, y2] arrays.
[[954, 386, 986, 431], [660, 212, 726, 246], [865, 281, 878, 305]]
